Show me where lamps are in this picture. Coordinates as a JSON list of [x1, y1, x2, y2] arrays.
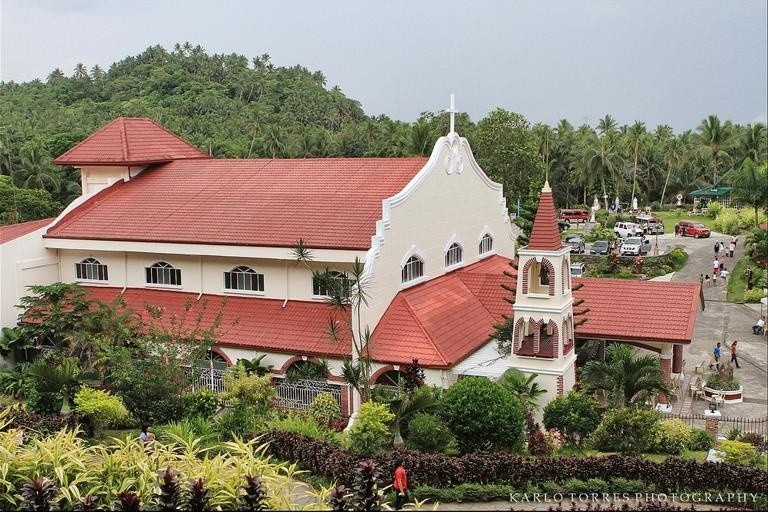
[[709, 402, 717, 413]]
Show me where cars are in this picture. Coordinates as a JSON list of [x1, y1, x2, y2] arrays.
[[620, 236, 649, 256], [613, 220, 644, 238], [590, 241, 612, 255], [636, 214, 665, 234], [675, 220, 711, 238], [562, 232, 585, 253]]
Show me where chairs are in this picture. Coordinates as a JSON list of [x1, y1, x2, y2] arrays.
[[690, 377, 706, 401], [695, 360, 705, 374], [670, 374, 684, 402], [758, 319, 768, 336], [712, 388, 726, 409]]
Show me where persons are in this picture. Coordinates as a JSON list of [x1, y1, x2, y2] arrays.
[[710, 342, 721, 369], [138, 422, 155, 455], [705, 275, 711, 288], [730, 340, 741, 368], [700, 274, 704, 286], [710, 233, 739, 285], [393, 457, 409, 510], [751, 316, 766, 335]]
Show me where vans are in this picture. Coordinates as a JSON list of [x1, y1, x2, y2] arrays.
[[559, 208, 591, 223]]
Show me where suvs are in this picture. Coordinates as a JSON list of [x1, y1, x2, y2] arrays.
[[570, 262, 586, 278]]
